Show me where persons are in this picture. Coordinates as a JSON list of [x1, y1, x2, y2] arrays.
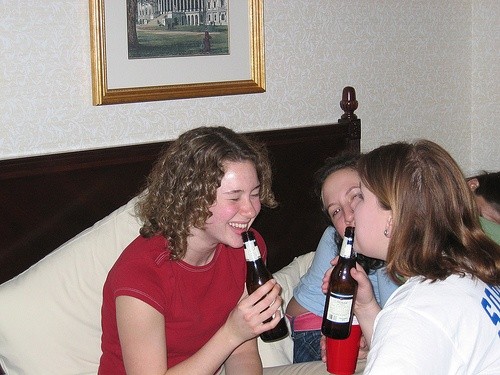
[[284, 140, 500, 375], [98, 127, 283, 374]]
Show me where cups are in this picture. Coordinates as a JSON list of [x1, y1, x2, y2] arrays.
[[326, 316, 362, 375]]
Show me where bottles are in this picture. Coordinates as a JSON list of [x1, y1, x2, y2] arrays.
[[241, 231, 289, 343], [321, 226, 357, 339]]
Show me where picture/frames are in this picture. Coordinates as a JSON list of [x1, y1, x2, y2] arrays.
[[89, 0, 266, 105]]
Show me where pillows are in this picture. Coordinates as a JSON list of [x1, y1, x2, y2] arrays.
[[0, 184, 155, 375]]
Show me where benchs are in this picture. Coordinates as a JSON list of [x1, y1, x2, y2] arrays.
[[0, 88, 362, 288]]
